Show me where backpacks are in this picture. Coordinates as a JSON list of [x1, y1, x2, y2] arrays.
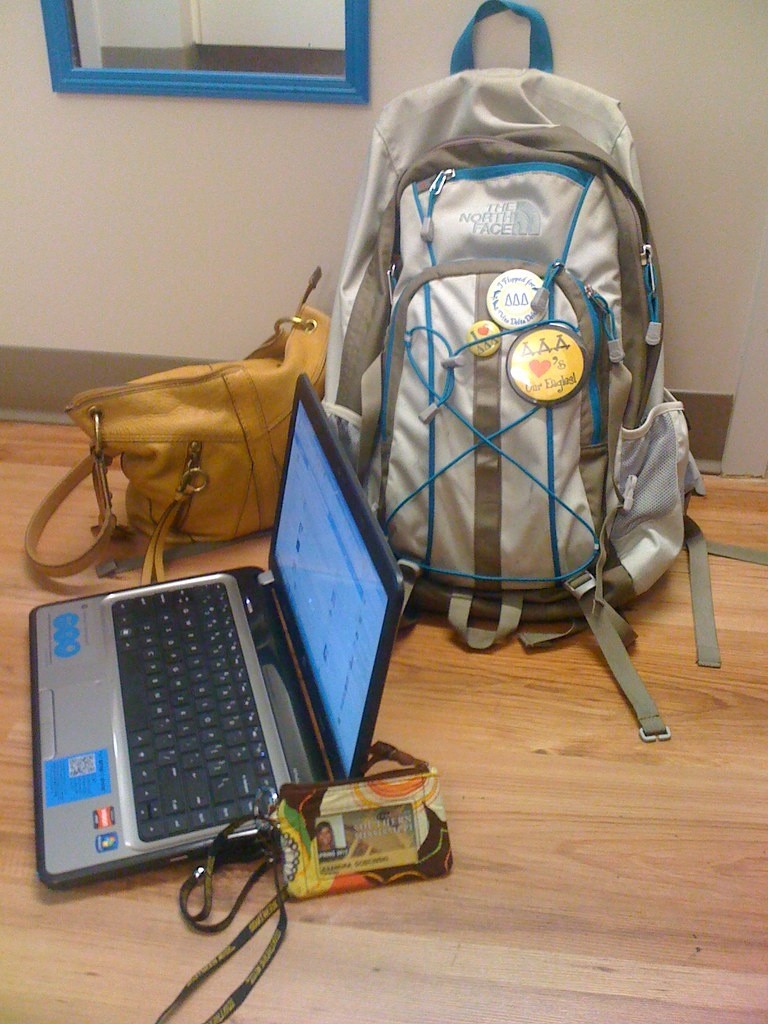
[[317, 0, 705, 650]]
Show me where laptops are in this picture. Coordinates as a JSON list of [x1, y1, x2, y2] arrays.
[[27, 373, 405, 894]]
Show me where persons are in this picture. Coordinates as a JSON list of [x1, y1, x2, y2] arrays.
[[316, 822, 335, 852]]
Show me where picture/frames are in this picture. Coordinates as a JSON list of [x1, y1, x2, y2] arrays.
[[40, 0, 370, 104]]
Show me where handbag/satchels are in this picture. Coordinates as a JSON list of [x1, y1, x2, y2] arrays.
[[22, 270, 332, 585], [156, 739, 455, 1023]]
